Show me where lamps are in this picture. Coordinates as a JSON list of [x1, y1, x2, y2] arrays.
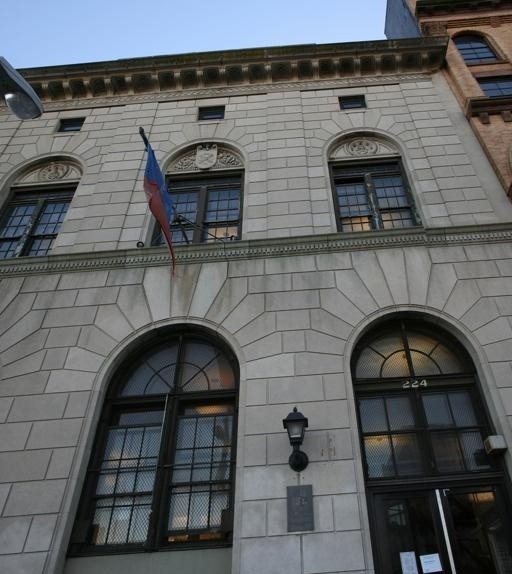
[[283, 406, 311, 473]]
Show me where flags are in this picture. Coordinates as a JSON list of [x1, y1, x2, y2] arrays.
[[142, 140, 176, 276]]
